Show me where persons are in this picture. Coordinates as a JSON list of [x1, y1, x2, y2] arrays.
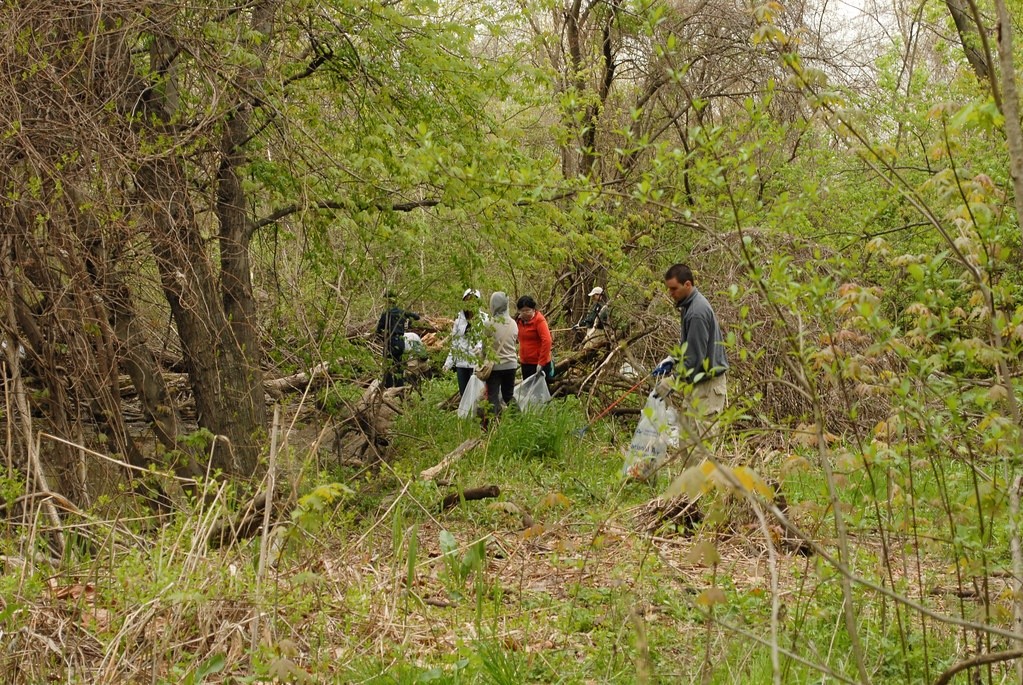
[[514, 295, 552, 393], [579, 286, 607, 350], [374, 291, 420, 399], [653, 264, 729, 470], [480, 291, 519, 418], [403, 318, 433, 380], [440, 287, 489, 419]]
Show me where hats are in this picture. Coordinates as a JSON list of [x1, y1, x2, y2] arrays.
[[588, 286, 605, 297], [383, 290, 399, 298]]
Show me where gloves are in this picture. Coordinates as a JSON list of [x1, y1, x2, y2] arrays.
[[535, 363, 544, 376], [651, 354, 676, 379], [653, 376, 679, 404]]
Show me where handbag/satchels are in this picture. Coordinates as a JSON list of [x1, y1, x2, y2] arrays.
[[475, 358, 496, 382]]
